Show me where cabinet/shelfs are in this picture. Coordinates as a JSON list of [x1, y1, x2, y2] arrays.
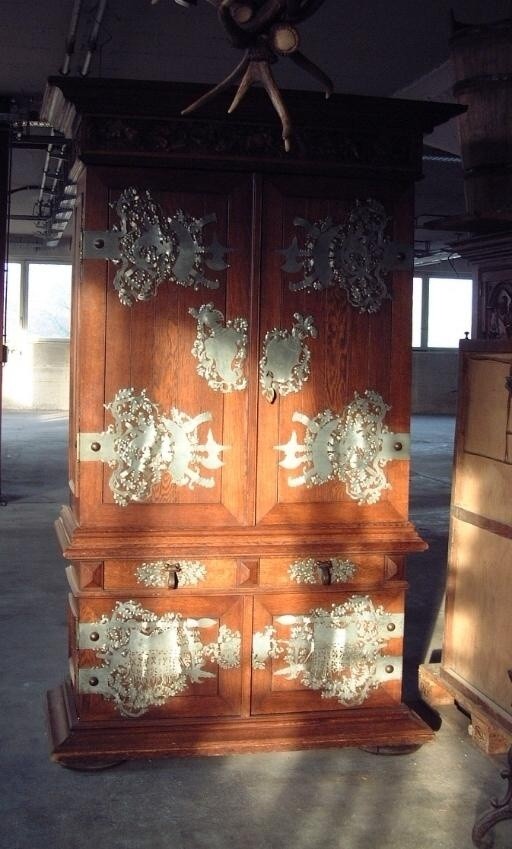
[[36, 70, 469, 774], [444, 354, 511, 719]]
